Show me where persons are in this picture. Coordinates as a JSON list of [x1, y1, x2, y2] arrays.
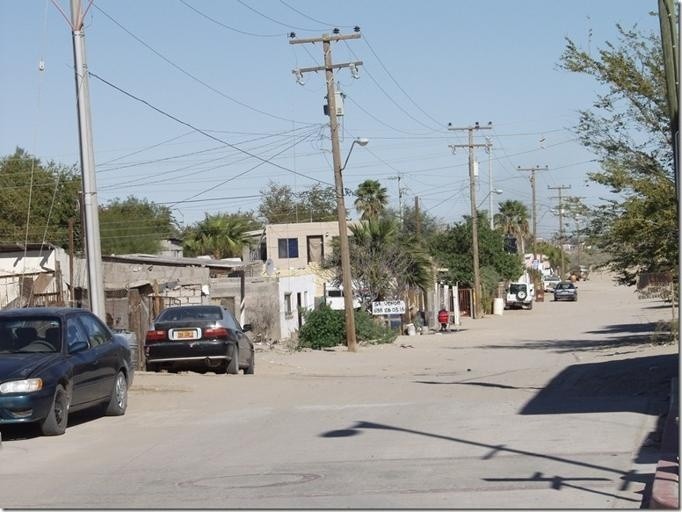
[[437, 305, 450, 332]]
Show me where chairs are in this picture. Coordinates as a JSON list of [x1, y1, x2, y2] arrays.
[[0, 328, 17, 350], [45, 327, 69, 352], [16, 327, 40, 350]]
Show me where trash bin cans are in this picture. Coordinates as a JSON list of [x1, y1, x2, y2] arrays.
[[571, 275, 576, 283], [115, 332, 138, 368], [493, 298, 503, 315]]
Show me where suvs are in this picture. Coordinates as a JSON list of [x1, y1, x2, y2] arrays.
[[505, 282, 534, 311], [542, 274, 562, 292]]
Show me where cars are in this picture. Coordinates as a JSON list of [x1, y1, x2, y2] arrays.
[[145, 304, 255, 376], [553, 282, 579, 302], [0, 307, 136, 435]]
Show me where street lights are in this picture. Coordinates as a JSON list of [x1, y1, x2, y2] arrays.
[[469, 187, 504, 318], [330, 136, 370, 351], [532, 208, 559, 297]]
